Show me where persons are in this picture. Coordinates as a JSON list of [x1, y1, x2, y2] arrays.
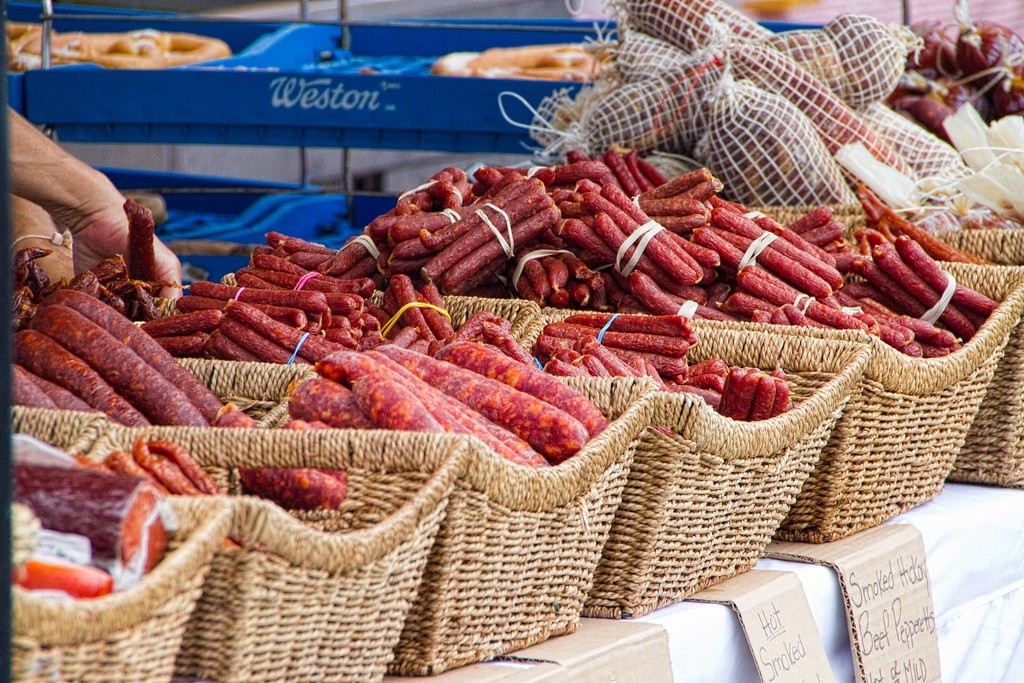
[[6, 104, 182, 311]]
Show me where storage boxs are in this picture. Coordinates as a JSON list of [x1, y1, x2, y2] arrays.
[[1, 0, 1024, 683]]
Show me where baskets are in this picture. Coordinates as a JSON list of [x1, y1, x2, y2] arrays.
[[915, 230, 1024, 490], [10, 497, 238, 682], [12, 401, 106, 468], [531, 308, 874, 619], [389, 375, 657, 678], [167, 357, 321, 433], [88, 424, 465, 683], [676, 259, 1024, 544]]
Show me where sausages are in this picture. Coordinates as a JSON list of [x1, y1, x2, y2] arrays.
[[10, 0, 1024, 600]]
